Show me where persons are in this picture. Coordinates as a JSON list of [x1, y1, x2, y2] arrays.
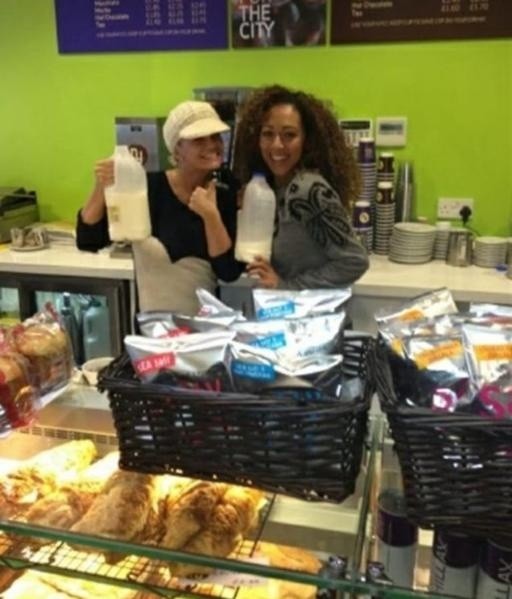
[[73, 97, 245, 342], [226, 83, 370, 299]]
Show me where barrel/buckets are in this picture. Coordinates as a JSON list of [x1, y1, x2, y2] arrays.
[[233, 170, 276, 264], [81, 301, 110, 358], [103, 143, 152, 242]]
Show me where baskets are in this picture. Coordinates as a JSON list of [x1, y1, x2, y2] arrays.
[[369, 344, 512, 536], [97, 335, 374, 502]]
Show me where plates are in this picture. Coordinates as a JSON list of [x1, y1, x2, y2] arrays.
[[387, 220, 511, 270]]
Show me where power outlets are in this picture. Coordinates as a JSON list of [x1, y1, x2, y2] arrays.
[[437, 198, 473, 218]]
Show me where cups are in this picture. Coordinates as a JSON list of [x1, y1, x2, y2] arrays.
[[350, 137, 414, 257]]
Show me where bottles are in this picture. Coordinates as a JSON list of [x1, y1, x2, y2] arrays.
[[60, 290, 84, 364]]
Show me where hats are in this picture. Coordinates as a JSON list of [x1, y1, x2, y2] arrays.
[[163, 101, 231, 153]]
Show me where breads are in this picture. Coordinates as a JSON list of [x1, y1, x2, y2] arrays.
[[2, 308, 75, 430], [2, 439, 321, 599]]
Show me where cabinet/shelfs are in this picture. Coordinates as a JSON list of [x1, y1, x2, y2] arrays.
[[0, 383, 512, 599]]
